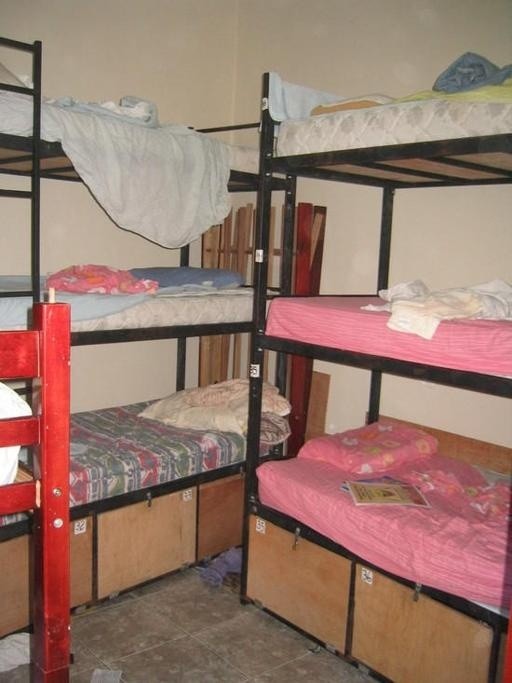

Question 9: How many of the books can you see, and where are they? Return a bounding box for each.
[341,476,421,495]
[345,483,431,507]
